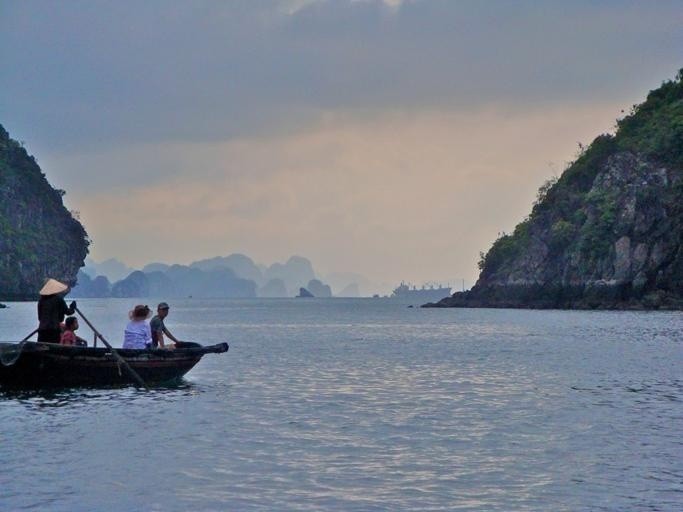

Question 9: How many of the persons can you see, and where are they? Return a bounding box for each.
[61,317,87,347]
[38,278,77,344]
[122,305,153,349]
[150,302,179,349]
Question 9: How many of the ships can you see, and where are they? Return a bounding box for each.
[393,282,452,299]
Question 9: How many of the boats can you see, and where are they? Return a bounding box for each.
[2,340,233,381]
[6,375,200,413]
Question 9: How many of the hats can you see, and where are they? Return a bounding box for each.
[38,278,68,296]
[158,302,170,310]
[128,305,153,322]
